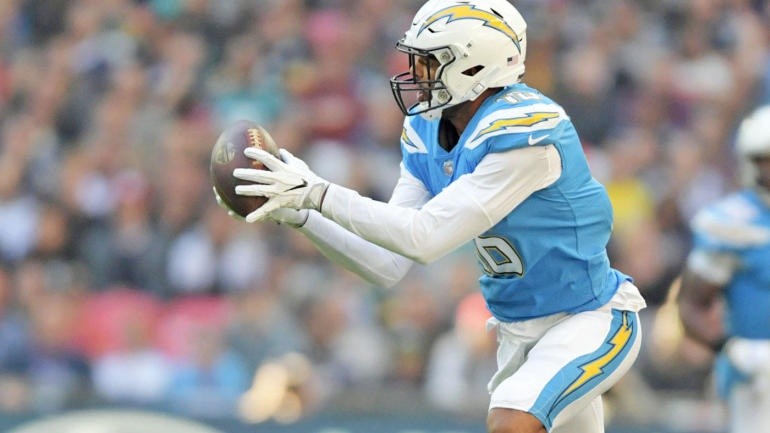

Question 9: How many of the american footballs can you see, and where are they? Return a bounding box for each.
[210,118,283,218]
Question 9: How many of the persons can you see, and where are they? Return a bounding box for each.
[0,1,504,427]
[509,0,769,432]
[212,0,647,433]
[677,105,769,431]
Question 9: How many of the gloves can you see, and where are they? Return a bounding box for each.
[214,181,311,228]
[233,143,332,224]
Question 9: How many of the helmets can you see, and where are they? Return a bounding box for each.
[389,0,526,116]
[734,105,770,191]
